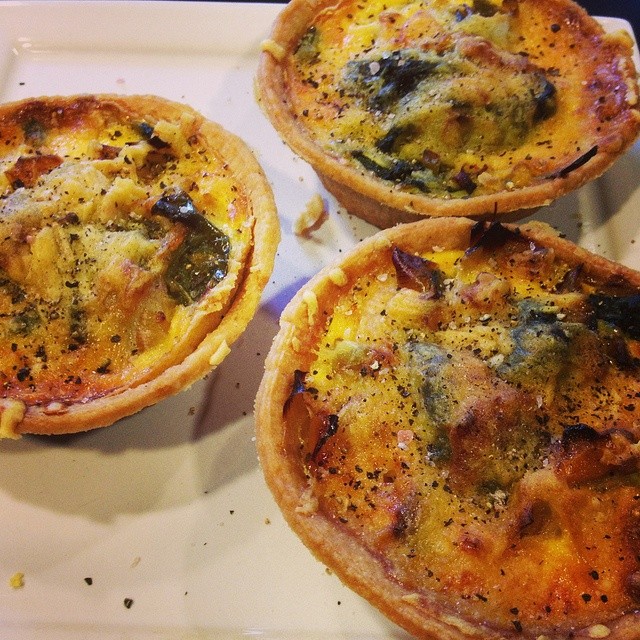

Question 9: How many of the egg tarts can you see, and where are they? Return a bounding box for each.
[250,1,640,232]
[0,92,282,438]
[253,215,640,639]
